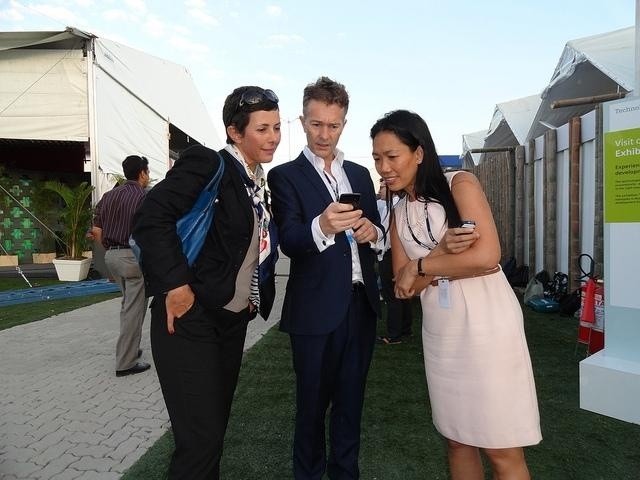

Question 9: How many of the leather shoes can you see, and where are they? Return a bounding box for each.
[115,361,151,378]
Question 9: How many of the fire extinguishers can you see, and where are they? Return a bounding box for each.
[574,254,604,353]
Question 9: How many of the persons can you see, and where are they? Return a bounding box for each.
[370,109,546,479]
[266,75,386,478]
[91,156,150,378]
[372,178,415,345]
[130,83,282,480]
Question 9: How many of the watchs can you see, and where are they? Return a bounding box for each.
[418,257,426,277]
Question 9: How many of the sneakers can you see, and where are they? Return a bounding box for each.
[137,348,143,358]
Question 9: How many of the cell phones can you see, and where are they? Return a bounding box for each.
[461,221,475,228]
[338,192,362,211]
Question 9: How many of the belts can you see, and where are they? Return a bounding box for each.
[108,243,132,250]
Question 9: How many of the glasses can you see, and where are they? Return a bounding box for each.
[228,87,281,125]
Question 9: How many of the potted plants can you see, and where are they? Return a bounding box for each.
[30,180,95,282]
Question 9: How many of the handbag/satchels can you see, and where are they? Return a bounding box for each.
[128,149,226,275]
